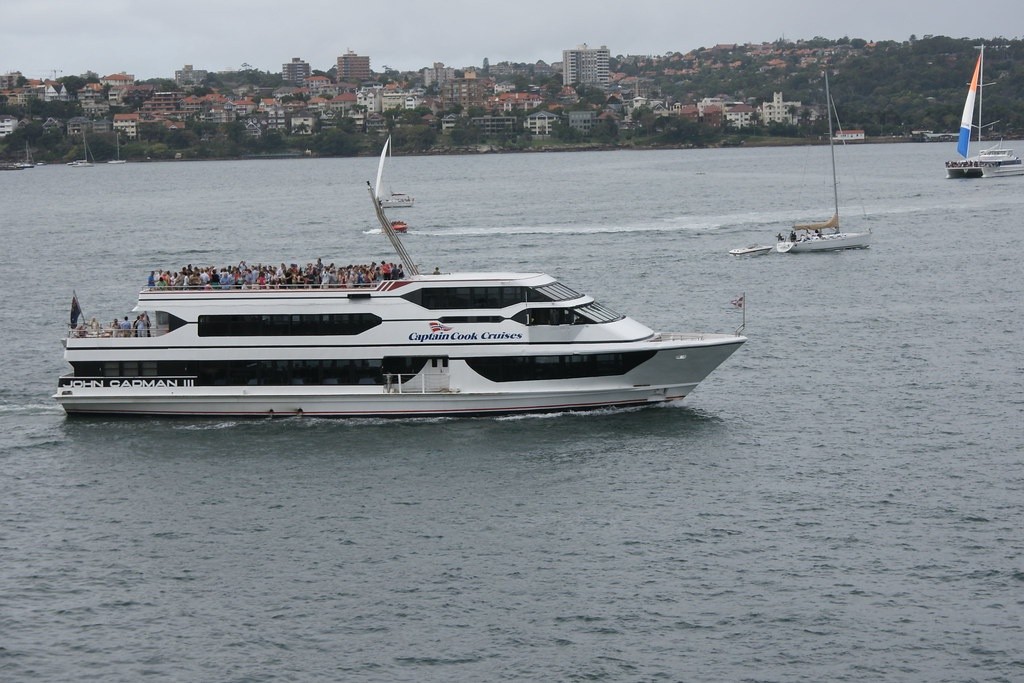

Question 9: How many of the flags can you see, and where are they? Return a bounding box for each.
[70,297,81,329]
[731,297,743,308]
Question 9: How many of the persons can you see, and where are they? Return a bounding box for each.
[433,267,440,275]
[148,258,405,290]
[112,313,151,336]
[945,161,978,167]
[79,317,99,336]
[790,230,796,242]
[806,229,819,236]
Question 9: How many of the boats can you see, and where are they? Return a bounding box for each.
[728,243,774,259]
[390,221,408,233]
[50,133,750,417]
[380,193,415,208]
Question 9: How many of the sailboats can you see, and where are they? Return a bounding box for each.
[945,44,1024,179]
[108,133,126,164]
[66,131,96,167]
[13,140,35,168]
[776,69,872,254]
